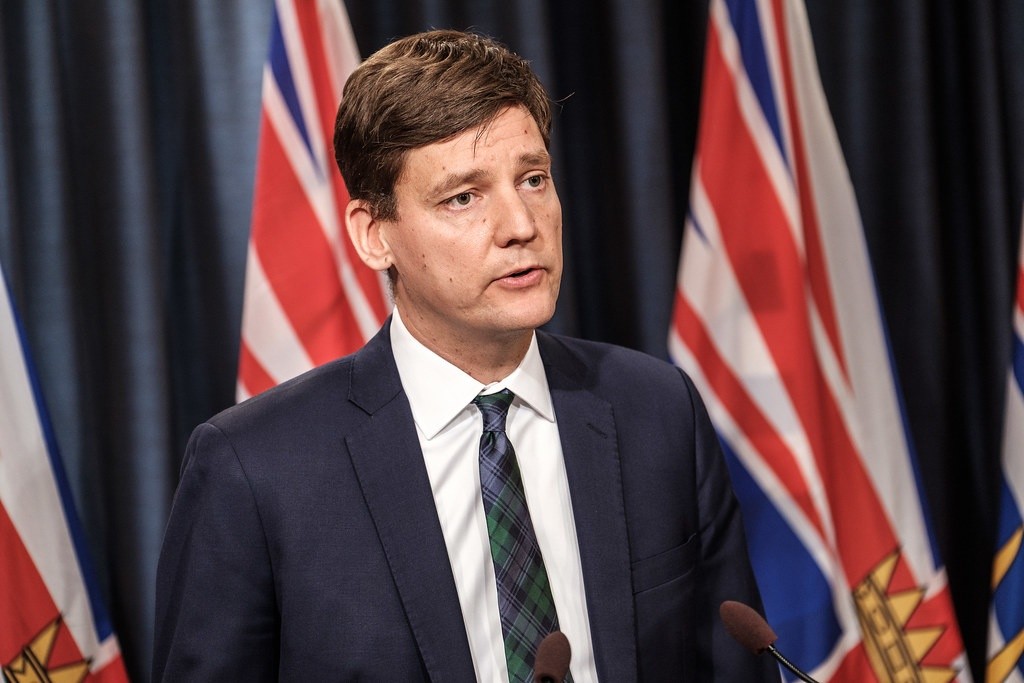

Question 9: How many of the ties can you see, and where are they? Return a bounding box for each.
[471,387,575,683]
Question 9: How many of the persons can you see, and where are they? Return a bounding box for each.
[153,32,767,683]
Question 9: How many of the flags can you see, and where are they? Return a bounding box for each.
[664,0,1024,683]
[0,266,133,683]
[233,0,403,403]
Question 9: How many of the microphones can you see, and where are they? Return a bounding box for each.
[535,631,571,683]
[720,601,817,683]
